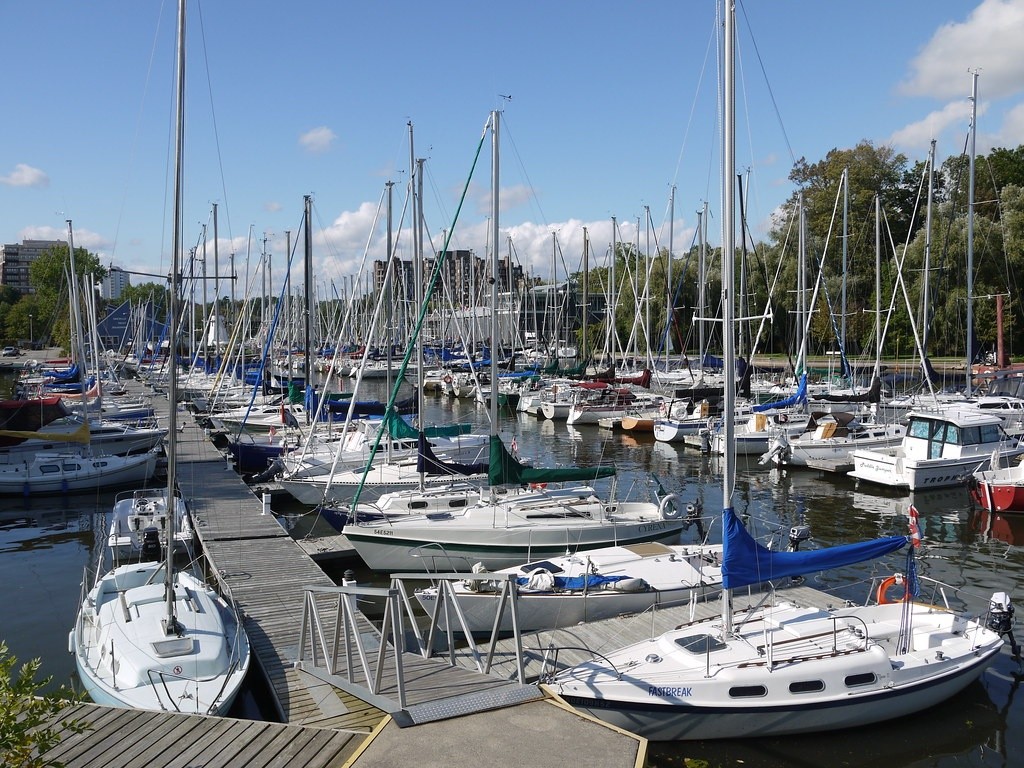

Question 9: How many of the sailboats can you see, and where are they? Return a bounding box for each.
[1,2,1024,754]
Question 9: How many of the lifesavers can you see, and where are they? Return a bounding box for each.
[876,574,912,605]
[660,495,683,519]
[532,482,547,489]
